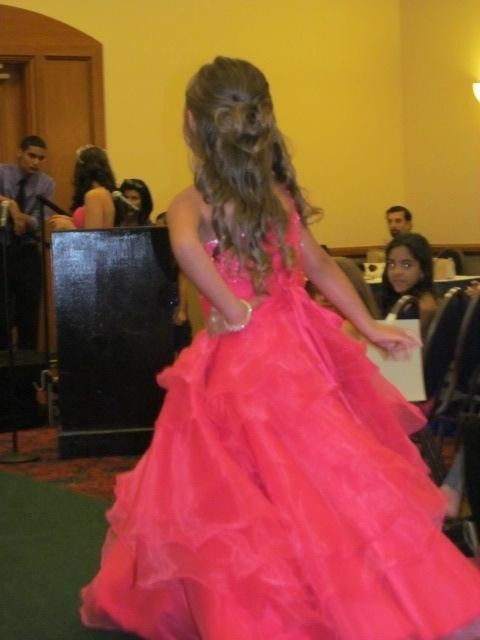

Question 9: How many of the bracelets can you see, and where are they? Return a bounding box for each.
[222,298,254,333]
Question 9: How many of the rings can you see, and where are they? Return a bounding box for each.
[211,314,216,323]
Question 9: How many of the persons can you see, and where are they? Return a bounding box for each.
[306,205,439,423]
[77,54,480,639]
[0,135,56,350]
[47,144,192,362]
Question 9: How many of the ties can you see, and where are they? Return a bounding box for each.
[14,180,26,214]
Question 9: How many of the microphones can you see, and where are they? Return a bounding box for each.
[111,190,140,213]
